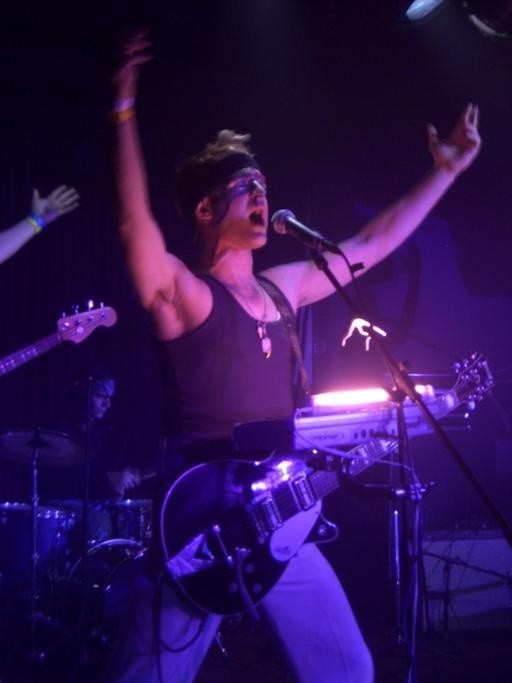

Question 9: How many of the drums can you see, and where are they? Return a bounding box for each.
[0,496,152,607]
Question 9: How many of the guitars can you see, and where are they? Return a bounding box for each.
[0,299,117,374]
[160,350,495,613]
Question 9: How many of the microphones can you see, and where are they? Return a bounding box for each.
[271,209,343,256]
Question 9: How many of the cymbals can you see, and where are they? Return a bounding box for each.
[1,429,79,462]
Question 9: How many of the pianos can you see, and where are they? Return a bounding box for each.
[230,391,471,454]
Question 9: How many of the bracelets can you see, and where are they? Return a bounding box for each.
[24,210,48,235]
[109,95,137,127]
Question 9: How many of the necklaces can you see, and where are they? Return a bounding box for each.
[230,278,277,360]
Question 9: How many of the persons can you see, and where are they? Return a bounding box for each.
[98,14,487,679]
[23,367,149,504]
[0,178,83,267]
